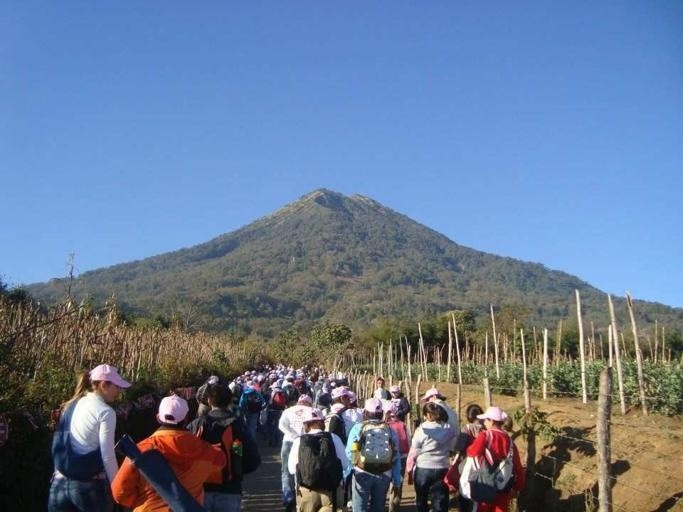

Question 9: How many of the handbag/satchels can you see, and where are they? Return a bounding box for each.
[492,456,514,490]
[53,402,103,478]
[459,455,497,501]
[328,414,346,444]
[444,460,461,494]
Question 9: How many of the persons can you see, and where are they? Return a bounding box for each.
[48,361,134,511]
[110,395,227,511]
[188,361,526,512]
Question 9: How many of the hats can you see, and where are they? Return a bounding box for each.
[159,394,190,425]
[89,363,132,389]
[476,407,507,422]
[299,386,402,422]
[422,389,439,401]
[233,365,344,388]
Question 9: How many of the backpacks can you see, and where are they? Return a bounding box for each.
[193,413,244,486]
[243,391,267,413]
[353,418,393,473]
[299,433,343,491]
[271,392,286,410]
